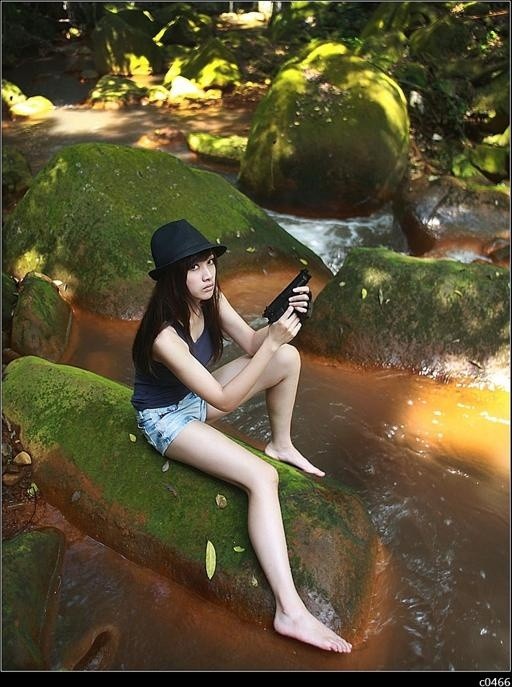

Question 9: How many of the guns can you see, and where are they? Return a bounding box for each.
[263,268,313,326]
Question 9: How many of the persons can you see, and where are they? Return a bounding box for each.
[130,218,354,655]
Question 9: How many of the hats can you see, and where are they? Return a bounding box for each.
[148,220,226,280]
